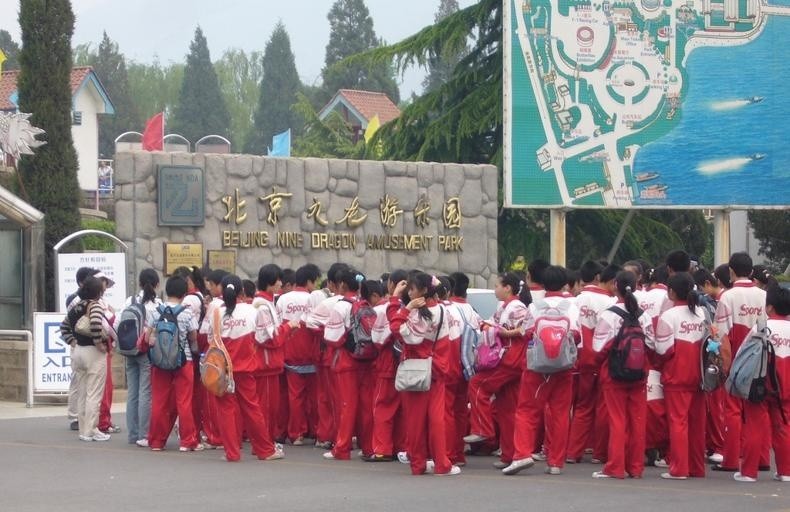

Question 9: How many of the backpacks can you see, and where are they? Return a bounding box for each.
[115,294,147,358]
[340,296,378,362]
[607,305,647,382]
[527,298,578,373]
[146,303,186,370]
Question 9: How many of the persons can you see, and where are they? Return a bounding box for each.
[99,160,113,193]
[114,263,479,474]
[463,251,790,482]
[59,267,121,442]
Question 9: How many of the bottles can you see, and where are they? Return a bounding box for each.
[199,353,205,364]
[526,341,536,351]
[707,364,720,374]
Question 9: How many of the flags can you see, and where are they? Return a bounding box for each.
[364,115,381,155]
[267,127,292,157]
[141,112,166,151]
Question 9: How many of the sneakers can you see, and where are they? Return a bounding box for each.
[136,430,304,460]
[493,445,790,482]
[315,433,486,475]
[71,421,120,442]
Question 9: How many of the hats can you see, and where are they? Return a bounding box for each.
[94,272,115,289]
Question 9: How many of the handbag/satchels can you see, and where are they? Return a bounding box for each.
[199,339,234,398]
[461,323,479,377]
[475,329,504,371]
[725,331,769,402]
[393,359,433,393]
[74,314,109,342]
[700,326,732,392]
